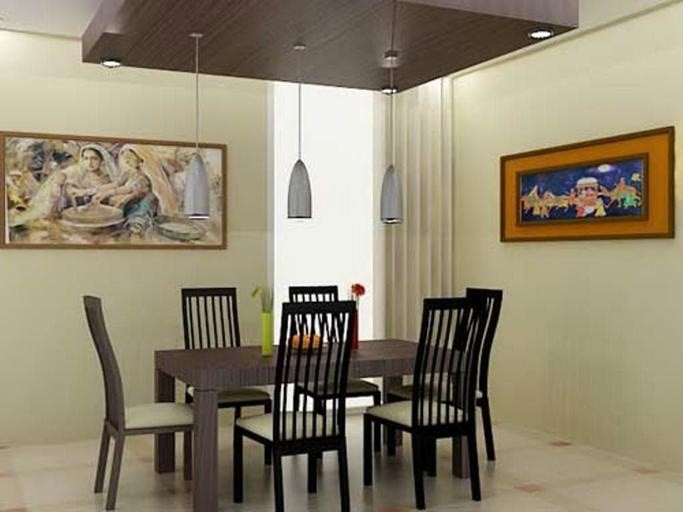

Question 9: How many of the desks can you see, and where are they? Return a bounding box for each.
[154,339,471,512]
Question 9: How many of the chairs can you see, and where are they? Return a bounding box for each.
[289,286,381,460]
[386,288,503,461]
[83,296,195,511]
[181,287,272,468]
[234,300,357,512]
[364,296,491,510]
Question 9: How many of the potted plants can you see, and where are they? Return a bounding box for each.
[251,284,272,356]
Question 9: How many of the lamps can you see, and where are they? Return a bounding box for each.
[185,32,209,218]
[381,53,403,223]
[288,44,312,219]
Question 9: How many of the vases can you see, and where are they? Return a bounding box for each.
[352,307,359,350]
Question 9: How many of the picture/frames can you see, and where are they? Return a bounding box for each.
[0,130,227,250]
[499,127,674,242]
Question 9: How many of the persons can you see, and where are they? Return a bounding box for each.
[3,135,223,247]
[522,174,644,218]
